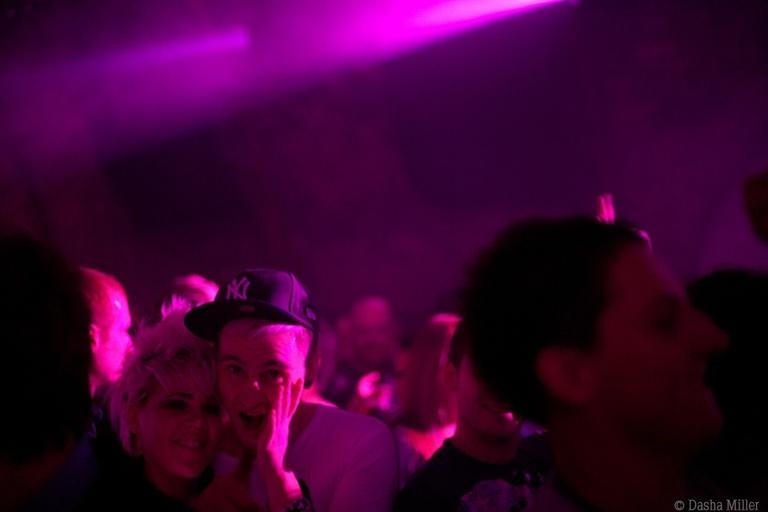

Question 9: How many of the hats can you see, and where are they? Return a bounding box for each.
[184,268,317,337]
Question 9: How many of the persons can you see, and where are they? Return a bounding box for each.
[0,169,768,512]
